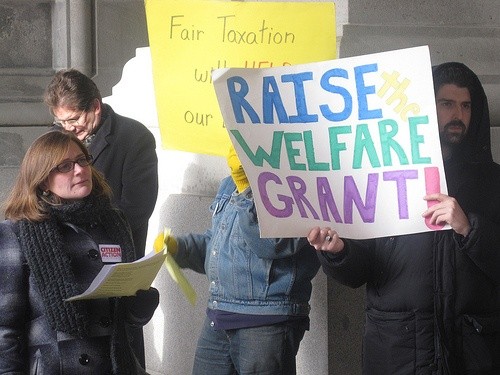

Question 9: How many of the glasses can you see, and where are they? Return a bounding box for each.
[53,108,86,127]
[53,154,93,173]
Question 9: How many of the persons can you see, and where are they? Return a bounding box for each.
[44,70,159,260]
[0,131,159,375]
[307,62,500,375]
[153,145,320,375]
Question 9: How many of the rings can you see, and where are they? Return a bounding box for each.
[325,236,332,242]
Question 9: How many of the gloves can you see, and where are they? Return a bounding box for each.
[154,233,177,260]
[227,143,250,193]
[131,287,159,318]
